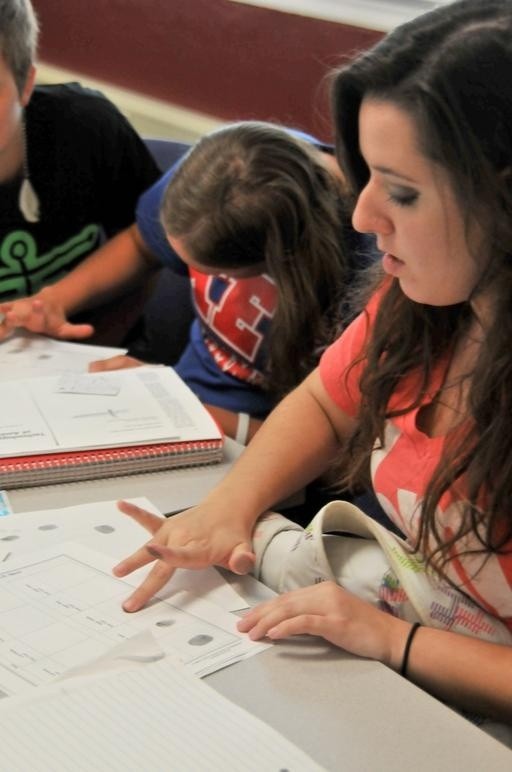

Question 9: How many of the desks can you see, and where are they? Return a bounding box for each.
[0,535,512,766]
[3,339,304,516]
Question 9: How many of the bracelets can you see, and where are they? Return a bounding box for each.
[400,623,426,674]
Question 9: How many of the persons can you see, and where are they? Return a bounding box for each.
[0,2,196,372]
[0,116,402,544]
[109,0,512,755]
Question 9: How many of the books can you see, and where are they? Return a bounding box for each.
[0,362,225,492]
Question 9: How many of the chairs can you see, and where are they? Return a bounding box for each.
[142,139,192,173]
